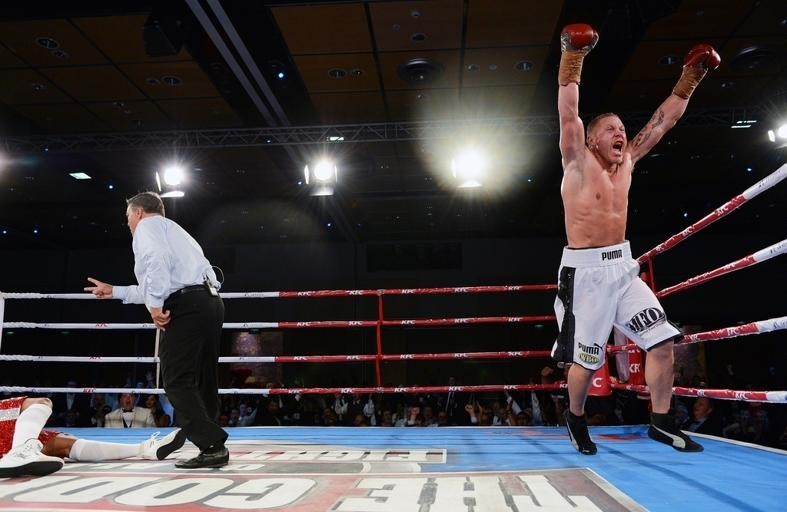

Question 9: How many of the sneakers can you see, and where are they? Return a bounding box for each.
[0,438,65,477]
[562,408,597,455]
[142,428,186,461]
[647,423,704,453]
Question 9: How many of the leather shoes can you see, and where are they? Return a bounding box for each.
[174,448,230,469]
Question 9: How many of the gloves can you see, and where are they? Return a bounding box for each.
[557,23,600,87]
[671,43,722,100]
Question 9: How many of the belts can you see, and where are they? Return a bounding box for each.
[176,287,209,295]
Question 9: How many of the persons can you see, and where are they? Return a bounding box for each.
[550,24,721,454]
[54,367,768,444]
[0,396,187,477]
[84,191,228,468]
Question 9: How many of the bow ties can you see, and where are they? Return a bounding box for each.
[123,409,133,413]
